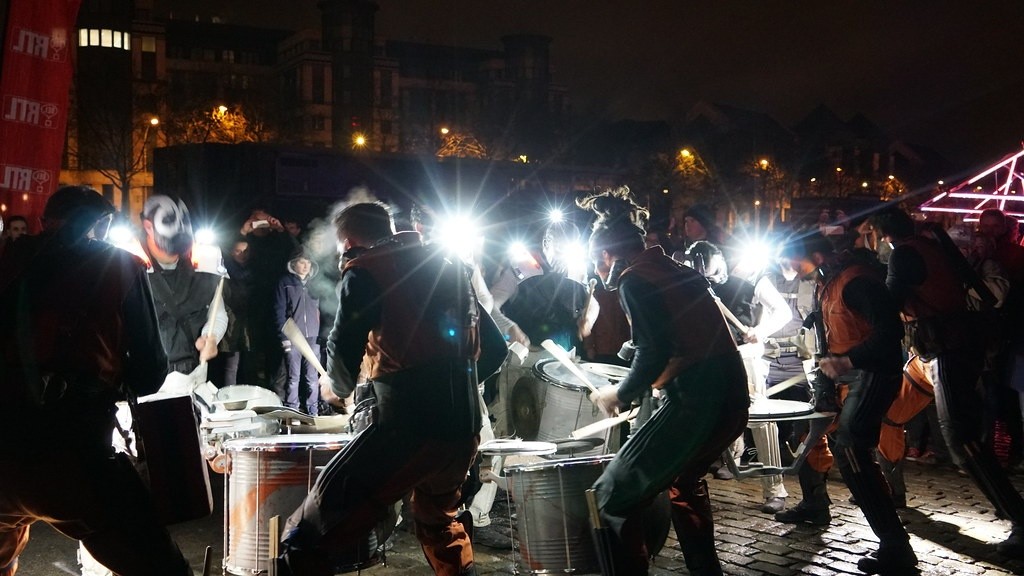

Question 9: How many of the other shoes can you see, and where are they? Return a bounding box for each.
[1014,460,1024,471]
[472,525,512,549]
[919,450,937,465]
[958,469,969,478]
[906,447,920,461]
[762,496,786,513]
[717,465,734,479]
[379,530,395,551]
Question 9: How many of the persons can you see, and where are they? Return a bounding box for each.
[863,204,1024,552]
[275,202,511,576]
[0,184,198,576]
[642,200,810,511]
[771,229,920,574]
[452,234,633,540]
[77,176,399,576]
[573,185,751,576]
[2,215,28,249]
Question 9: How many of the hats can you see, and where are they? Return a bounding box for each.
[684,202,714,232]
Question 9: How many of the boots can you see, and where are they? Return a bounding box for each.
[592,524,640,576]
[841,459,918,573]
[775,469,832,525]
[849,449,906,508]
[960,446,1024,556]
[671,496,724,576]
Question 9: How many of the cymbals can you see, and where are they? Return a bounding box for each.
[580,362,632,381]
[511,376,541,442]
[250,405,316,425]
[549,436,606,456]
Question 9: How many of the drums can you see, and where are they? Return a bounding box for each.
[528,356,615,460]
[223,432,386,576]
[503,454,612,576]
